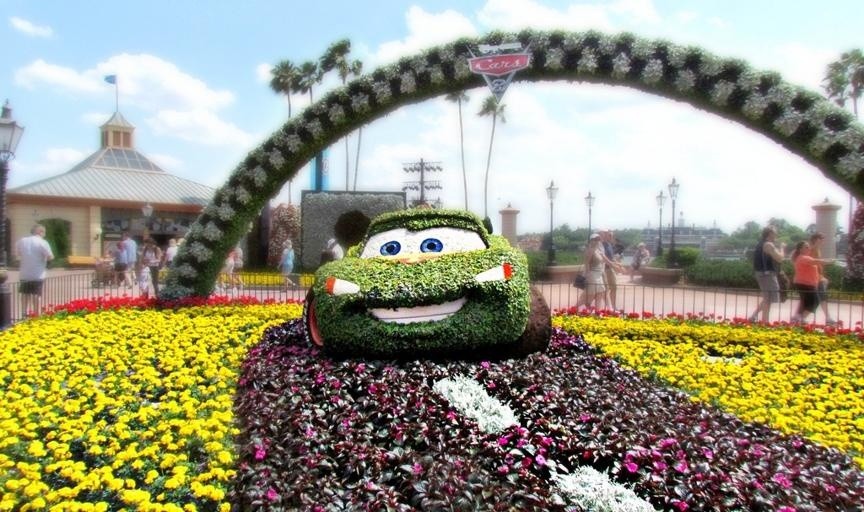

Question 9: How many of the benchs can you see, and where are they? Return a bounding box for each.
[66,255,99,271]
[639,266,684,285]
[546,264,586,284]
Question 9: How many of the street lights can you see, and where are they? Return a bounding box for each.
[544,177,561,266]
[0,97,27,336]
[667,177,680,269]
[140,199,154,242]
[656,189,667,259]
[584,190,596,248]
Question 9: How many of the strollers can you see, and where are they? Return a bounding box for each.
[90,250,117,291]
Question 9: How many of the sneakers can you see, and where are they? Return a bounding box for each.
[827,320,837,326]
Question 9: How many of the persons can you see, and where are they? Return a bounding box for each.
[807,231,837,327]
[326,238,345,259]
[597,226,627,313]
[571,234,622,309]
[14,224,57,315]
[97,224,246,299]
[749,225,785,324]
[277,240,297,293]
[790,240,838,324]
[631,241,649,271]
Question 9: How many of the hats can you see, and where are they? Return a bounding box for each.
[590,234,600,240]
[637,242,646,247]
[327,239,335,249]
[283,240,292,248]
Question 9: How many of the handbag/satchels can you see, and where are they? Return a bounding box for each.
[777,272,789,301]
[573,275,586,290]
[818,282,827,301]
